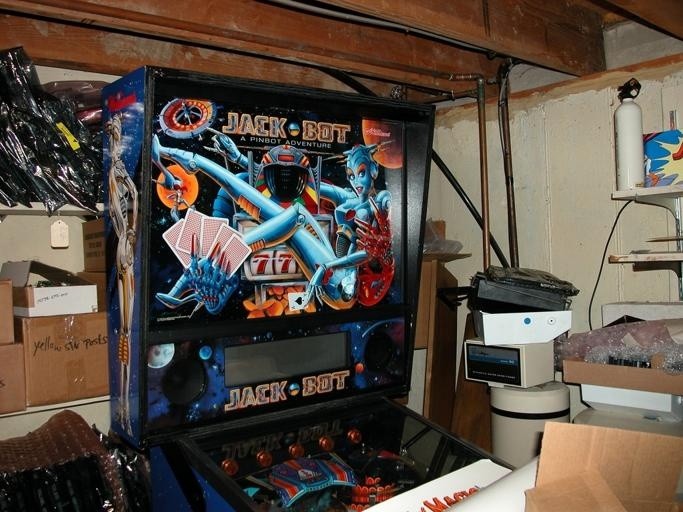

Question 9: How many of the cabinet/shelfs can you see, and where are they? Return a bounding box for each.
[0,56,438,417]
[602,185,683,327]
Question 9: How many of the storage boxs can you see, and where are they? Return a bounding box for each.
[523,317,683,512]
[0,217,123,413]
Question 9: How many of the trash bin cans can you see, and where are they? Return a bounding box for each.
[490,381,570,470]
